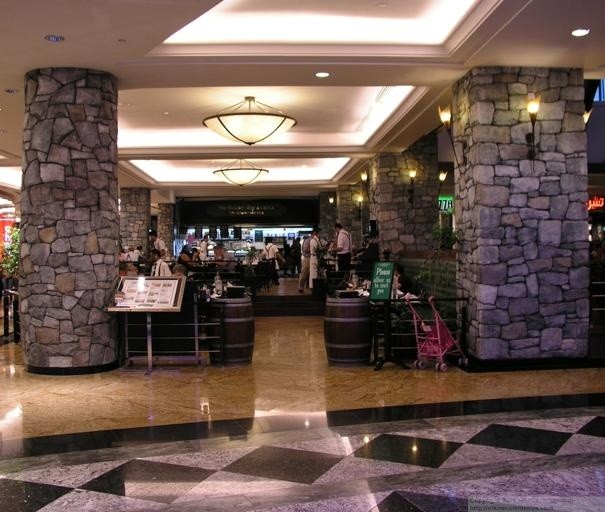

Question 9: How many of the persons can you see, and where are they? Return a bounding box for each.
[289,223,352,292]
[119,230,235,279]
[262,237,285,285]
[0,263,18,316]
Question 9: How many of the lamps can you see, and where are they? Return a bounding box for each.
[212,159,270,186]
[361,174,371,200]
[437,104,466,175]
[409,170,417,185]
[526,96,541,161]
[328,195,337,209]
[357,196,362,219]
[439,168,448,187]
[201,95,299,146]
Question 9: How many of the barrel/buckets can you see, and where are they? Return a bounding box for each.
[324,298,372,368]
[208,298,254,365]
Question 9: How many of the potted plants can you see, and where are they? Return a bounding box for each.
[0,226,21,289]
[312,246,327,300]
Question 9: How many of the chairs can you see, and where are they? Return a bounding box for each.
[119,260,274,293]
